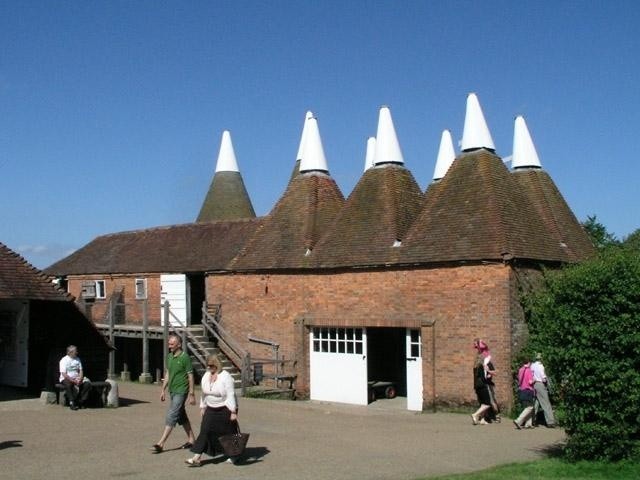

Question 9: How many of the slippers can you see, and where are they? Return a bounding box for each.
[149,444,164,453]
[184,458,201,467]
[180,441,193,449]
[225,457,235,464]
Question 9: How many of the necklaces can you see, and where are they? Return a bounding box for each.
[210,373,217,391]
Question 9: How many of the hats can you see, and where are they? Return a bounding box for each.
[535,352,543,360]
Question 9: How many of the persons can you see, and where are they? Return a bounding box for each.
[472,339,492,379]
[59,344,91,411]
[471,351,500,426]
[530,352,556,430]
[183,352,244,466]
[509,355,537,429]
[480,352,498,420]
[147,334,196,452]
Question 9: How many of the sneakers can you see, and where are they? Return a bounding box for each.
[524,425,535,429]
[547,424,556,428]
[513,420,522,429]
[78,401,85,409]
[470,413,479,425]
[478,420,490,425]
[69,402,77,410]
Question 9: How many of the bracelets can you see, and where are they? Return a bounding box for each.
[231,411,237,414]
[190,392,195,396]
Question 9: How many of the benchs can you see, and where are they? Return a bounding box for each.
[54,379,119,407]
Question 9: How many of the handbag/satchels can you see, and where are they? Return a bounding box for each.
[218,419,250,457]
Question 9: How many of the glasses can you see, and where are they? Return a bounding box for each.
[207,364,214,368]
